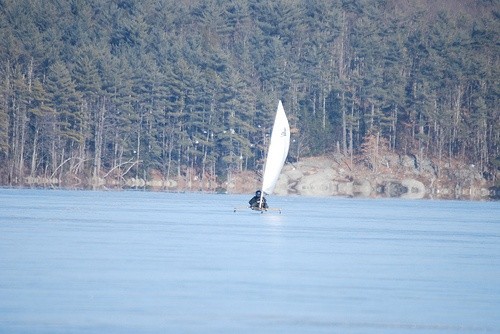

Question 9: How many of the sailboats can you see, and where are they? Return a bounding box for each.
[253,101,291,211]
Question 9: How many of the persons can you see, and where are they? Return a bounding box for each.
[249,190,269,208]
